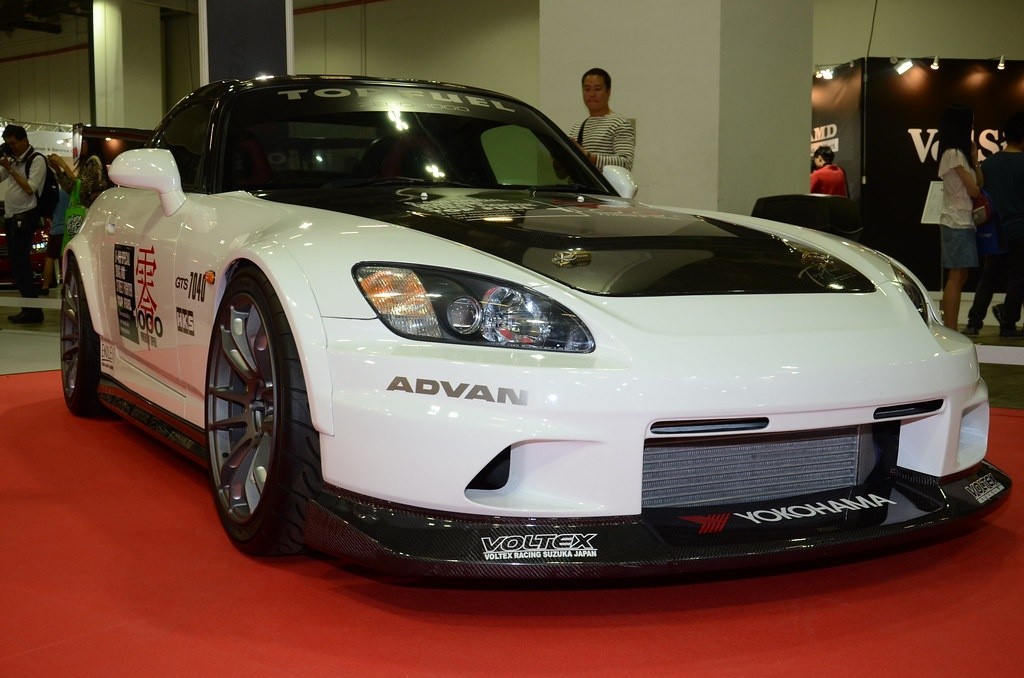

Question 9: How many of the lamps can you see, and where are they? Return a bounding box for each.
[930,55,940,70]
[814,63,841,79]
[890,56,915,75]
[993,55,1006,70]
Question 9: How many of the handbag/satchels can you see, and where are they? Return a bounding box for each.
[58,179,88,254]
[973,193,991,225]
[976,189,1000,257]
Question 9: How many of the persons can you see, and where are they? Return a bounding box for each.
[0,124,109,325]
[939,106,1024,337]
[550,68,636,193]
[811,147,849,197]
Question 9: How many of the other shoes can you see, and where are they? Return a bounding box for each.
[39,288,49,296]
[959,326,981,337]
[992,303,1018,337]
[6,310,44,324]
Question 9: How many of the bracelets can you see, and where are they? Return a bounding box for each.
[7,168,15,174]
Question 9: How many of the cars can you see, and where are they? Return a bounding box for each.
[61,74,1011,584]
[0,200,61,288]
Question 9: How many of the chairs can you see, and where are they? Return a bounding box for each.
[349,135,444,178]
[222,130,273,187]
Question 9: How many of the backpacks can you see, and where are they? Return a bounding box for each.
[24,151,60,216]
